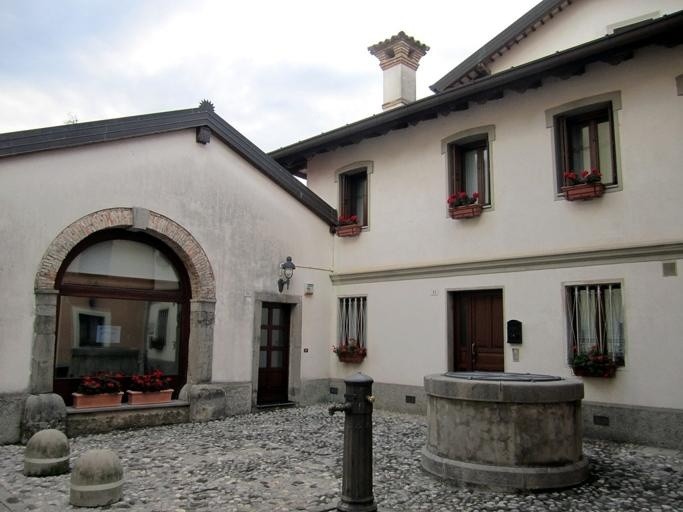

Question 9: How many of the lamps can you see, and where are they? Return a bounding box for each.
[278,257,295,295]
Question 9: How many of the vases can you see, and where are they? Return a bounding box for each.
[333,225,361,237]
[123,383,174,406]
[71,390,124,408]
[335,353,365,365]
[573,366,618,378]
[557,183,606,200]
[448,204,483,219]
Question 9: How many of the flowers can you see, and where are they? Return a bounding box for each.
[335,212,360,227]
[126,368,170,391]
[571,344,626,368]
[446,191,479,208]
[562,168,606,183]
[73,369,125,396]
[332,335,365,356]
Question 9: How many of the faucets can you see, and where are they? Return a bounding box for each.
[328,402,349,417]
